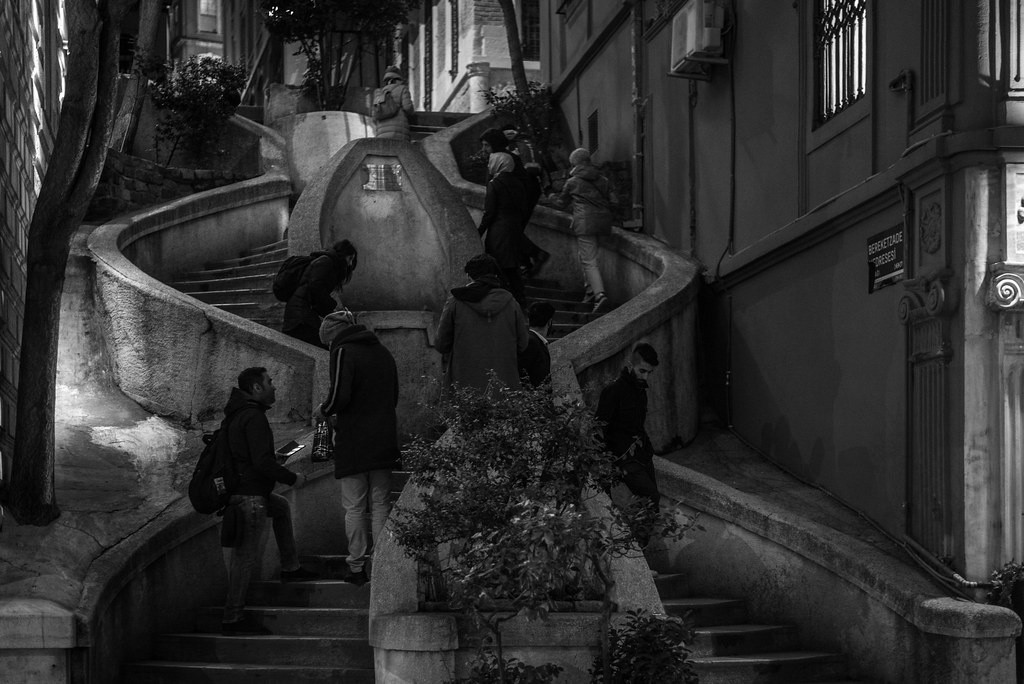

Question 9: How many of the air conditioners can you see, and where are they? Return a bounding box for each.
[670,1,733,78]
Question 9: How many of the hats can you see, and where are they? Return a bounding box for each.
[319,310,355,342]
[383,65,404,80]
[480,128,510,153]
[569,148,590,165]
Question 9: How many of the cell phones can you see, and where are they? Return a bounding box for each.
[284,444,306,457]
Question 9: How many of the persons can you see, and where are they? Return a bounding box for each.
[374,65,416,140]
[516,301,556,411]
[216,365,320,637]
[594,342,663,578]
[282,239,358,350]
[477,152,528,310]
[506,141,549,277]
[434,251,530,407]
[548,149,615,314]
[312,308,399,587]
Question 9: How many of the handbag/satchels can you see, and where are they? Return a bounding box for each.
[311,416,333,463]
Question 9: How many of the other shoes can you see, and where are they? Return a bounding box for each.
[221,622,255,637]
[344,569,369,582]
[581,290,595,303]
[591,294,608,314]
[280,566,318,582]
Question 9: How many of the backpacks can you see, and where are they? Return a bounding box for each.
[188,404,262,515]
[373,84,405,121]
[272,255,336,303]
